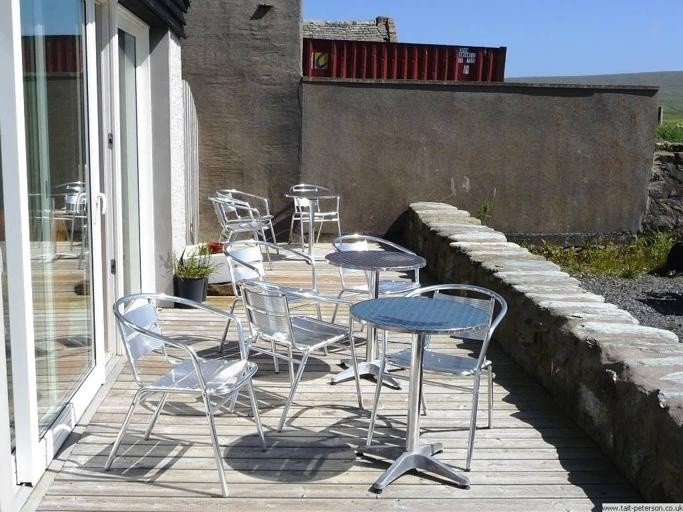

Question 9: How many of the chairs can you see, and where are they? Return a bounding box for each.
[27,180,86,271]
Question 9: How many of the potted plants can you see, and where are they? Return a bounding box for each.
[74,280,90,295]
